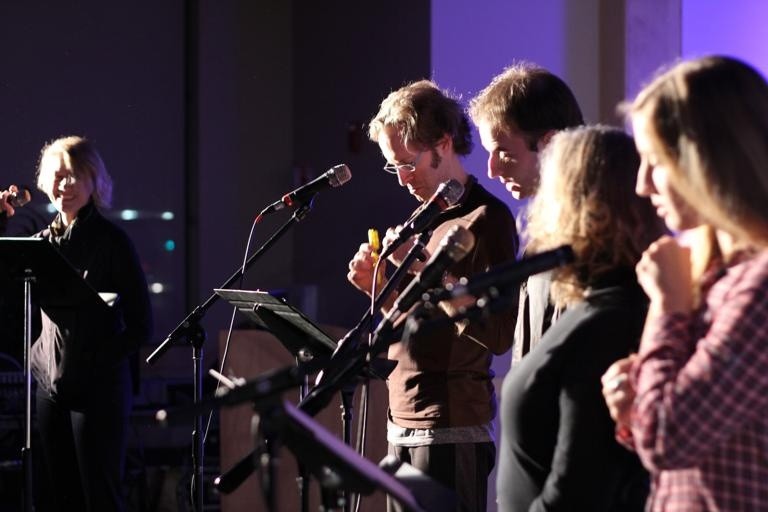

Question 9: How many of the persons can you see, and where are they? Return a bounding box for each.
[346,80,520,512]
[600,55,768,512]
[467,62,585,366]
[0,136,154,512]
[496,125,674,512]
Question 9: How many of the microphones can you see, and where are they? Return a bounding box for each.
[375,223,474,334]
[442,243,576,303]
[8,189,30,209]
[379,177,466,260]
[262,164,353,214]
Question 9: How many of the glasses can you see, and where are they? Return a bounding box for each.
[383,146,427,175]
